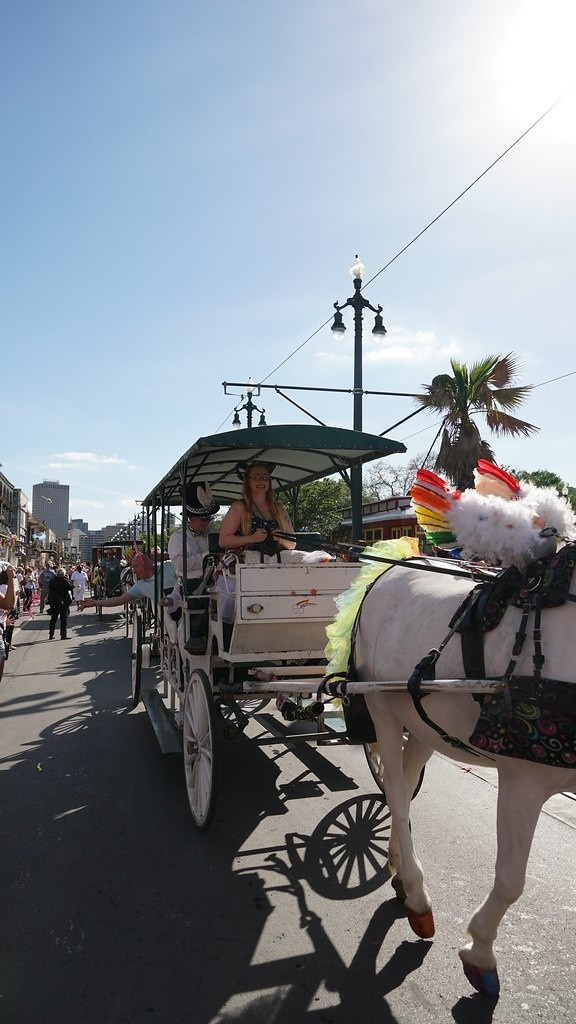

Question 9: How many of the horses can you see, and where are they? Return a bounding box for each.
[352,548,576,995]
[120,566,140,624]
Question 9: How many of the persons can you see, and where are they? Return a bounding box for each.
[168,514,213,637]
[160,577,183,626]
[100,549,119,576]
[0,561,94,682]
[77,553,178,611]
[217,464,331,625]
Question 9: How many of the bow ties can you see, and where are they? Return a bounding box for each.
[193,532,206,538]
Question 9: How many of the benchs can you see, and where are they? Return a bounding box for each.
[206,531,322,651]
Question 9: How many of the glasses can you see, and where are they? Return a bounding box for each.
[198,515,210,522]
[248,476,269,481]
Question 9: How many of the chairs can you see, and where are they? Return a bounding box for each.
[164,586,183,689]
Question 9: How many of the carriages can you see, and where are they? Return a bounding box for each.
[88,540,141,624]
[130,422,576,998]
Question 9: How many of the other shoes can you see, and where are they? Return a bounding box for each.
[61,636,71,640]
[49,637,55,640]
[7,644,17,650]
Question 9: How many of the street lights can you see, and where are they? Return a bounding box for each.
[232,376,268,429]
[331,254,388,548]
[109,505,147,557]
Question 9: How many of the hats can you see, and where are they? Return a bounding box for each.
[179,481,220,517]
[28,569,33,572]
